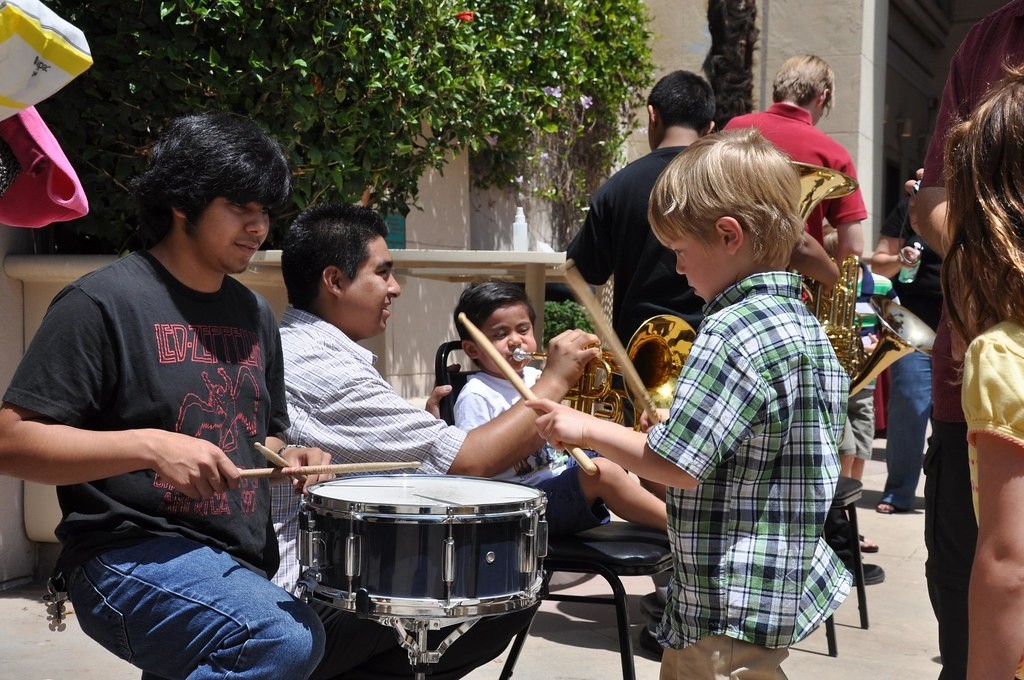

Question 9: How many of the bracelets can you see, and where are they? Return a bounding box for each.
[278,445,306,458]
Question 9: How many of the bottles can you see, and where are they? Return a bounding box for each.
[898,242,921,283]
[512,207,528,251]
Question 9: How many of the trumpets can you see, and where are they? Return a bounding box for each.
[510,312,701,462]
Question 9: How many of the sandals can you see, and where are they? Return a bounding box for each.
[876,501,900,514]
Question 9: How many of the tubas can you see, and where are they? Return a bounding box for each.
[784,156,936,421]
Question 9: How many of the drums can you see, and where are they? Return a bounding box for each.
[293,474,547,621]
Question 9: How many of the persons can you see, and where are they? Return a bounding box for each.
[871,196,942,513]
[0,110,540,680]
[942,64,1024,680]
[453,277,669,537]
[824,224,886,587]
[545,69,841,428]
[515,128,854,680]
[272,201,600,593]
[0,114,76,228]
[721,54,867,262]
[908,1,1023,680]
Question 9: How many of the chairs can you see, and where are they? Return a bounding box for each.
[821,477,868,657]
[434,341,672,680]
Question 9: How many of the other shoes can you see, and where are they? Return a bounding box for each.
[859,538,879,553]
[639,628,664,655]
[843,563,884,585]
